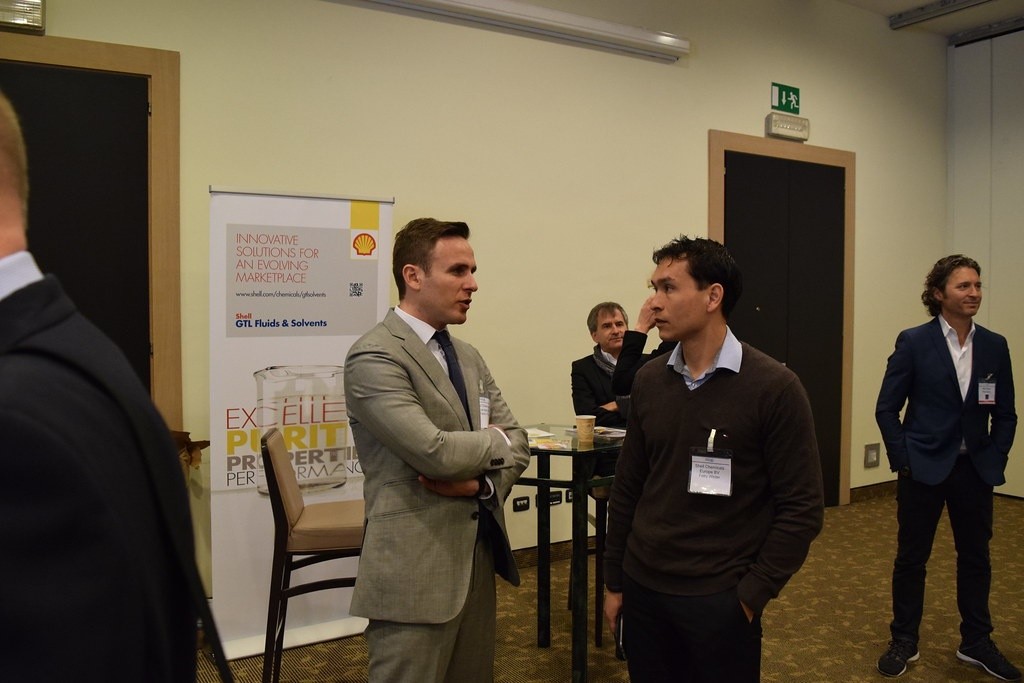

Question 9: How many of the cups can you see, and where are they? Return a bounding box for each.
[576,415,596,443]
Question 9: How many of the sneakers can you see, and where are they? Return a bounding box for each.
[877,640,920,677]
[956,639,1022,681]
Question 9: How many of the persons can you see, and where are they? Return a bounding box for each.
[569,236,826,682]
[875,253,1024,683]
[341,218,532,683]
[2,95,206,683]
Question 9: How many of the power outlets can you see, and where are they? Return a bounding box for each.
[865,443,880,467]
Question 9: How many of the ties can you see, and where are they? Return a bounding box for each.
[431,330,474,431]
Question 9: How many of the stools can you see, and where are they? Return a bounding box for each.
[257,428,365,683]
[568,478,611,648]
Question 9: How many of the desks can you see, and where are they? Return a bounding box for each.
[520,424,627,683]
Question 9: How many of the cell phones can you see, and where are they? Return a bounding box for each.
[620,614,627,661]
